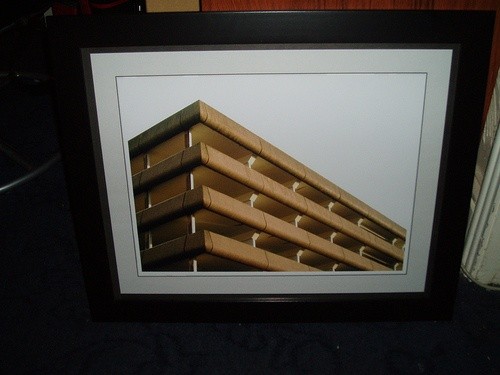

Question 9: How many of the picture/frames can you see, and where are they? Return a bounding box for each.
[43,7,497,326]
